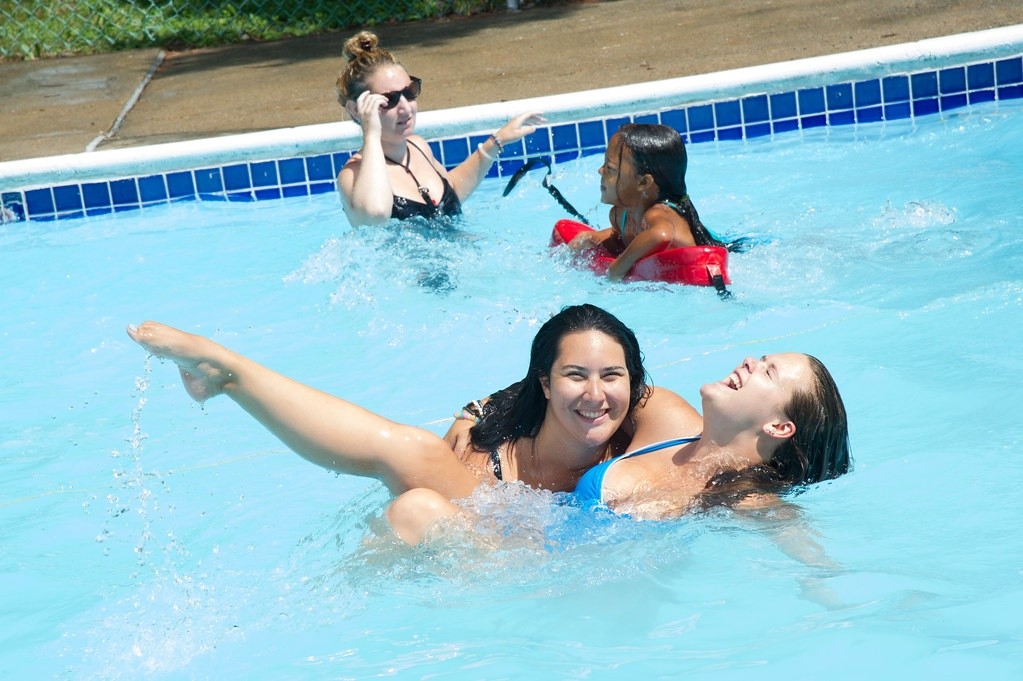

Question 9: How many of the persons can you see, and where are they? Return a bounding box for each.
[568,123,714,276]
[126,322,850,547]
[335,30,547,229]
[442,303,655,492]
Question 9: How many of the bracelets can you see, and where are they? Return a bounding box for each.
[478,143,496,161]
[491,134,504,153]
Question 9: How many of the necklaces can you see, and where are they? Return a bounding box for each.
[385,146,435,211]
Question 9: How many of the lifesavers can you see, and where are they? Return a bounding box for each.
[548,218,733,287]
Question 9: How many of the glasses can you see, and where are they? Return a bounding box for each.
[378,75,422,110]
